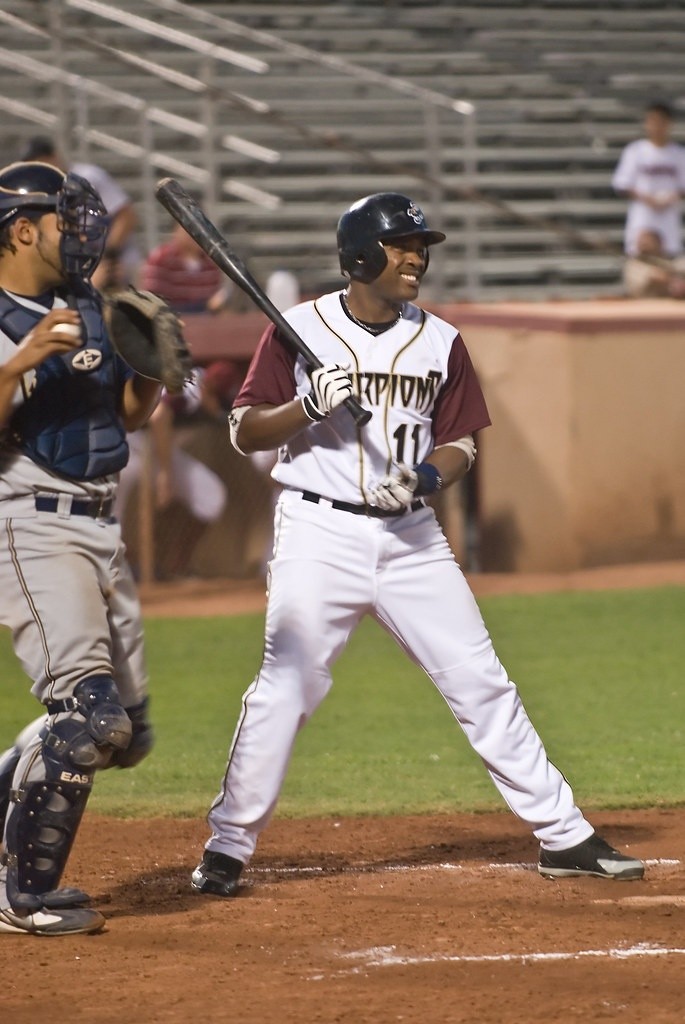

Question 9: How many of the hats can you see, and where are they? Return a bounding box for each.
[22,136,56,161]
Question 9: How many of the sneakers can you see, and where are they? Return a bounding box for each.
[0,904,106,935]
[538,832,645,881]
[191,849,244,896]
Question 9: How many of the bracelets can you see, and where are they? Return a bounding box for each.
[412,463,442,496]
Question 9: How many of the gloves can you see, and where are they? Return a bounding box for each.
[301,363,356,422]
[364,461,442,511]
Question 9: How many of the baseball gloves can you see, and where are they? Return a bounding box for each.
[104,284,198,389]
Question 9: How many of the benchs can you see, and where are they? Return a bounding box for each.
[0,0,685,303]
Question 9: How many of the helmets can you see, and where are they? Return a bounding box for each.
[0,161,86,222]
[337,193,446,284]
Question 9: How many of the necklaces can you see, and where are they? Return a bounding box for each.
[343,290,402,333]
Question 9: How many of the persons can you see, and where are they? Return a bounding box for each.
[0,161,165,937]
[72,163,278,533]
[612,102,685,298]
[190,192,645,898]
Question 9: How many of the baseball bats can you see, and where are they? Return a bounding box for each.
[152,177,372,428]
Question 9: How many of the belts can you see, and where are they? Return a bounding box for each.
[35,496,117,519]
[301,490,424,518]
